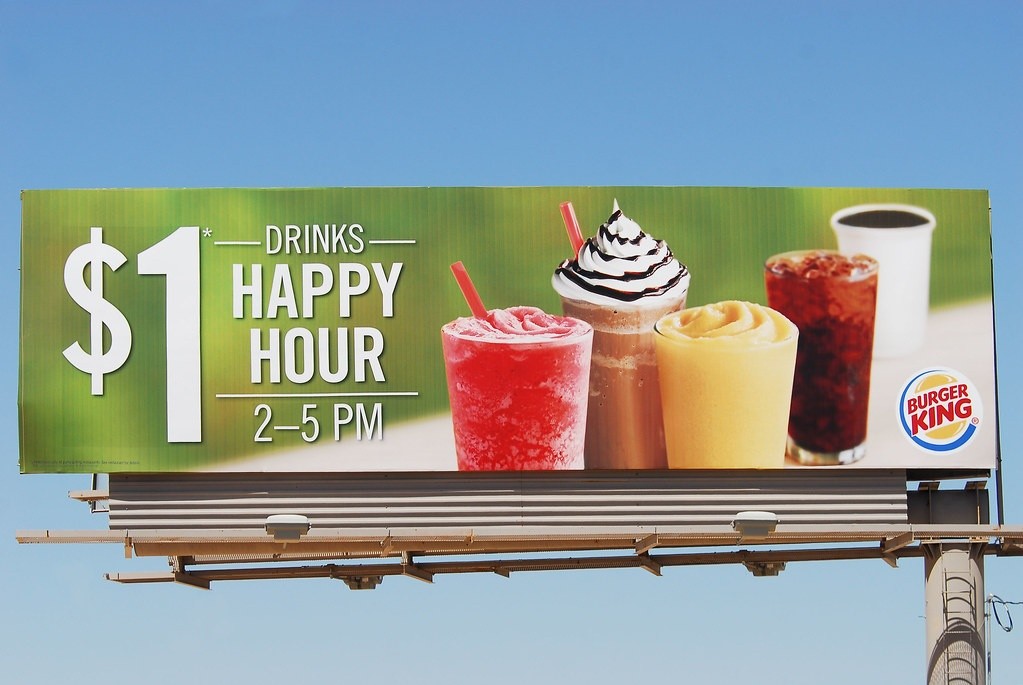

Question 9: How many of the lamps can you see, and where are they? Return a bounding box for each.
[343,575,384,590]
[263,514,312,544]
[744,561,787,577]
[731,511,780,539]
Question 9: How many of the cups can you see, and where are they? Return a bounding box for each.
[560,289,687,469]
[654,311,799,469]
[441,317,594,470]
[831,204,936,356]
[765,250,879,466]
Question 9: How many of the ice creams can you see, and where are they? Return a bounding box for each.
[550,196,691,308]
